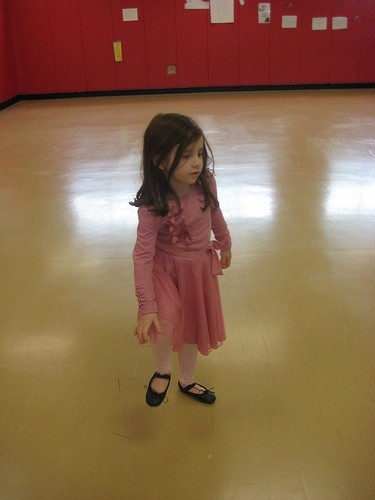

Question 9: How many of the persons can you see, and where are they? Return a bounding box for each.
[132,113,231,406]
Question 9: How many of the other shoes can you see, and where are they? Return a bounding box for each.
[145,369,172,406]
[177,378,216,404]
